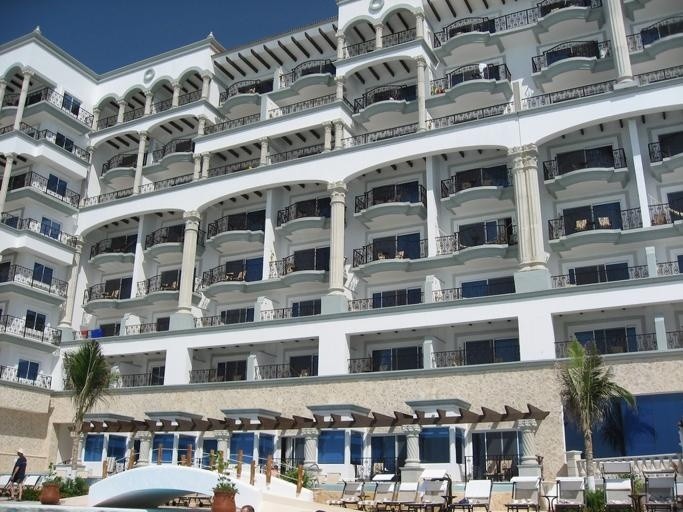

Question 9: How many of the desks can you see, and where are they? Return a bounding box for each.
[627,493,646,511]
[442,495,456,509]
[539,495,558,511]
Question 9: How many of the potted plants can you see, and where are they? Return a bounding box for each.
[211,472,238,512]
[37,473,59,505]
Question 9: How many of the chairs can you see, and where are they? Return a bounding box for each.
[357,479,398,511]
[602,477,636,511]
[553,480,587,511]
[642,470,679,511]
[504,480,539,511]
[382,482,420,511]
[404,477,453,511]
[448,479,493,511]
[0,473,56,500]
[326,477,366,509]
[82,213,683,302]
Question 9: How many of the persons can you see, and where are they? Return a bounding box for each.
[8,448,27,500]
[240,504,255,512]
